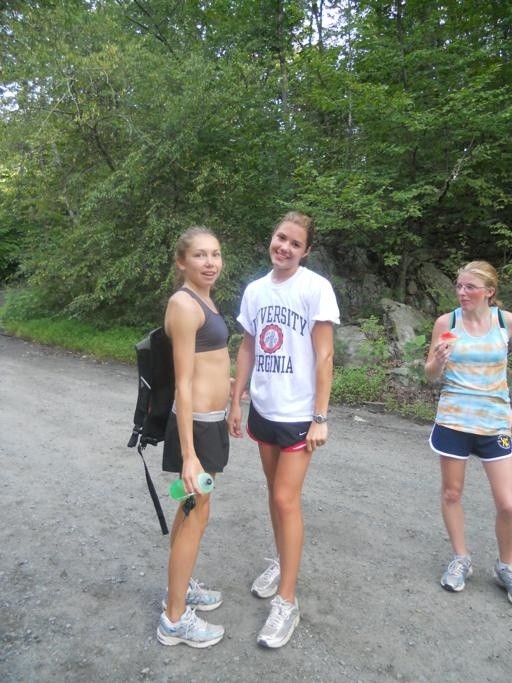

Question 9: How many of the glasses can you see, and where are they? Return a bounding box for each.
[455,282,490,292]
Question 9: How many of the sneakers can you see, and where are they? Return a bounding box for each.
[155,604,227,650]
[161,576,223,612]
[439,554,474,592]
[492,556,512,604]
[249,556,282,599]
[256,594,300,650]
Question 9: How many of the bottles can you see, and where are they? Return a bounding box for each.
[169,472,215,502]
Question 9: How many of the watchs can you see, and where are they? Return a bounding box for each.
[312,414,328,423]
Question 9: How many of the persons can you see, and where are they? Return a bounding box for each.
[226,209,340,648]
[424,260,512,605]
[156,226,248,646]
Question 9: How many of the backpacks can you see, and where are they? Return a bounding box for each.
[127,325,168,453]
